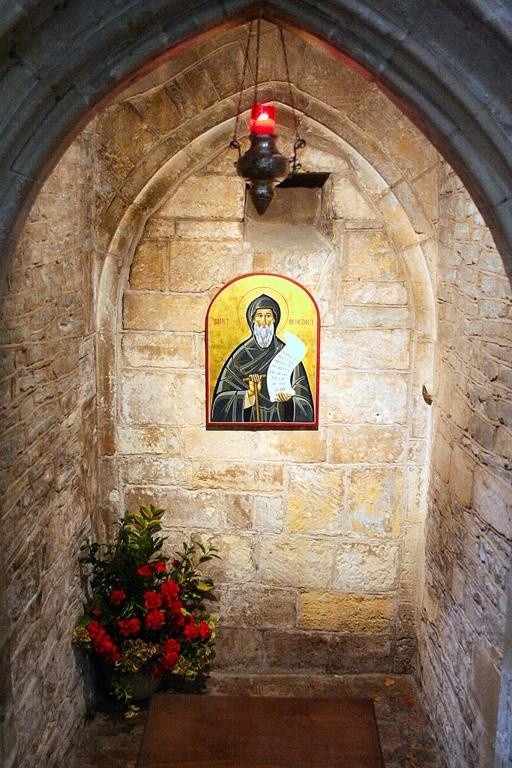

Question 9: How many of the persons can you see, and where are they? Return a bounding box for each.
[212,292,315,423]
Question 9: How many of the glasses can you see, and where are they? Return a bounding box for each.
[229,0,306,217]
